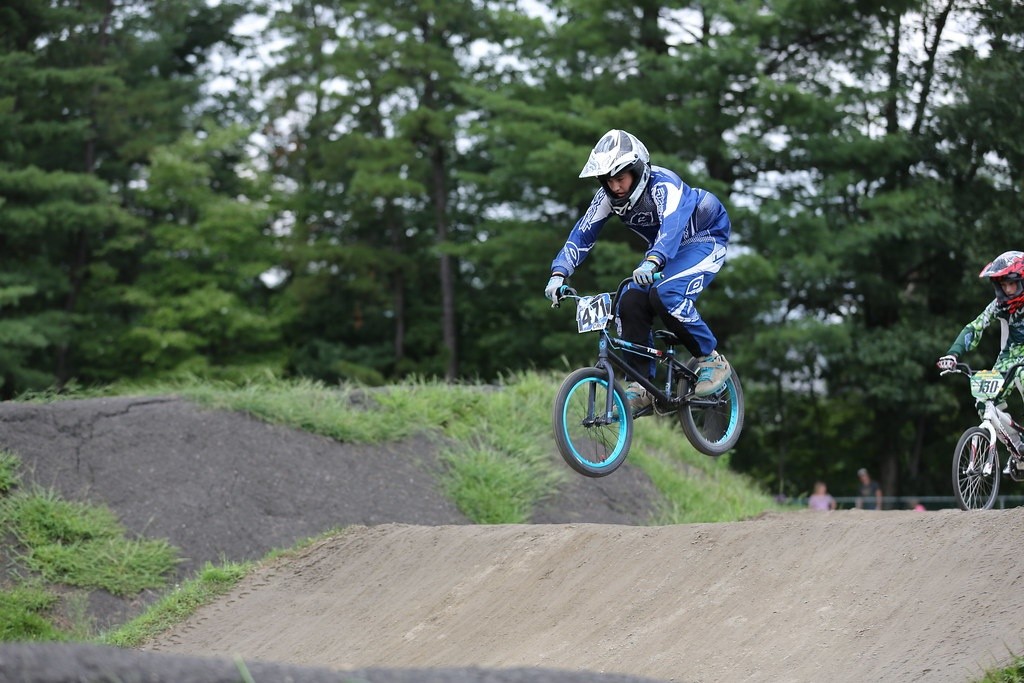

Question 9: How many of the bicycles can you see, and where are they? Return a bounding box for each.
[939,358,1024,511]
[552,271,744,478]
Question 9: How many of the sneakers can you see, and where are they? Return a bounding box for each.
[695,353,732,397]
[609,380,656,418]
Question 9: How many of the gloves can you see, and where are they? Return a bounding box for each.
[545,274,565,308]
[633,260,659,285]
[936,354,957,371]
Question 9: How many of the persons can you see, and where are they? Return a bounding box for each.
[936,248,1024,476]
[544,127,732,422]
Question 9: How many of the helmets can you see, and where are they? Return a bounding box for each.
[578,129,651,216]
[979,251,1024,314]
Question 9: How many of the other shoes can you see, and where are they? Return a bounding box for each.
[1002,453,1016,474]
